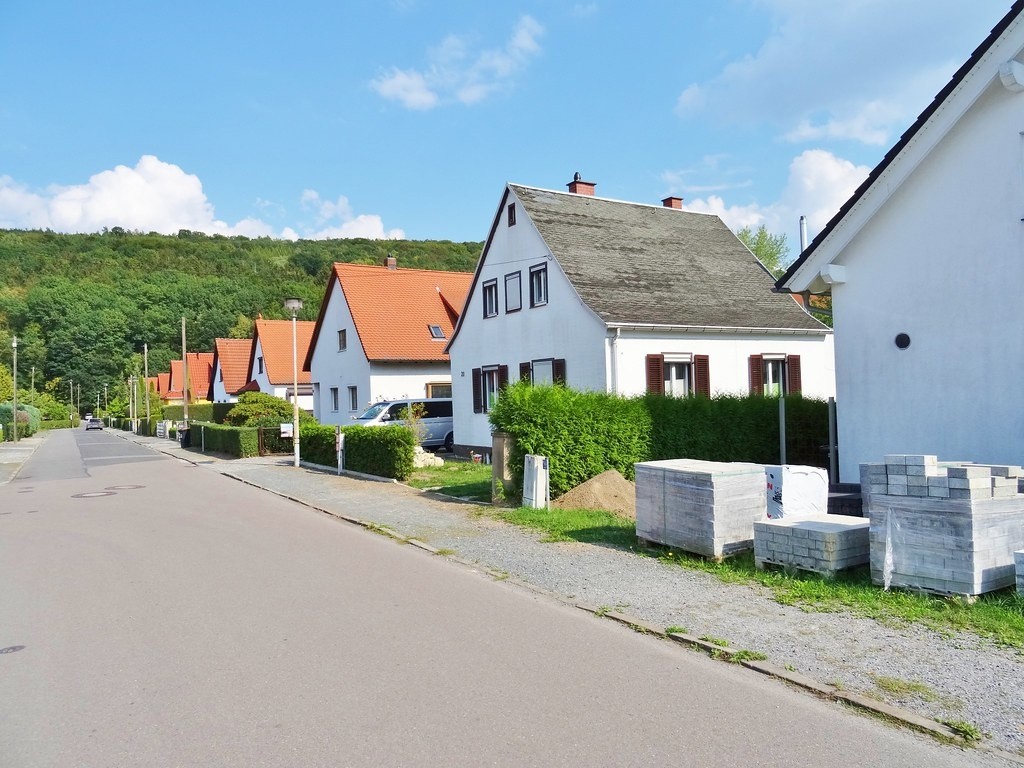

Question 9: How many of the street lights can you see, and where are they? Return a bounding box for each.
[283,296,304,467]
[103,383,108,416]
[76,384,81,413]
[69,380,73,419]
[12,341,18,442]
[96,391,100,417]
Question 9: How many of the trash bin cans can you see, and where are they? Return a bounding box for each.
[178,428,191,449]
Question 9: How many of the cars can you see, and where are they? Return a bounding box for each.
[86,418,104,430]
[84,413,93,420]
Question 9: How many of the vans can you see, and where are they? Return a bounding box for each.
[344,398,453,452]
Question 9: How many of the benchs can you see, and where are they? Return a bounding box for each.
[157,420,172,439]
